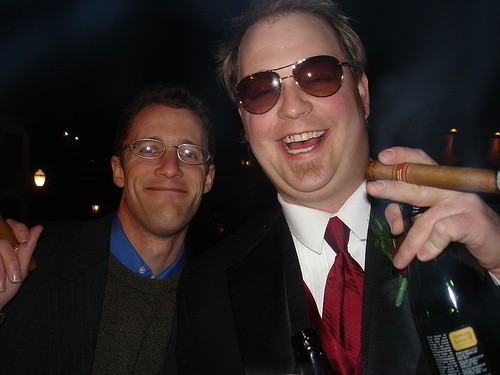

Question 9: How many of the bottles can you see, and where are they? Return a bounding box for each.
[400,202,500,375]
[290,328,338,375]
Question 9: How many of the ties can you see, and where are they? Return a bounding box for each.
[321,216,364,375]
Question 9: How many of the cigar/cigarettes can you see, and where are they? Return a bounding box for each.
[365,159,500,196]
[0,214,38,272]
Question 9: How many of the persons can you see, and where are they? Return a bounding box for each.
[176,1,500,375]
[0,85,214,375]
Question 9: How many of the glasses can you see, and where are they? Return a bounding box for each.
[235,55,357,115]
[121,139,213,165]
